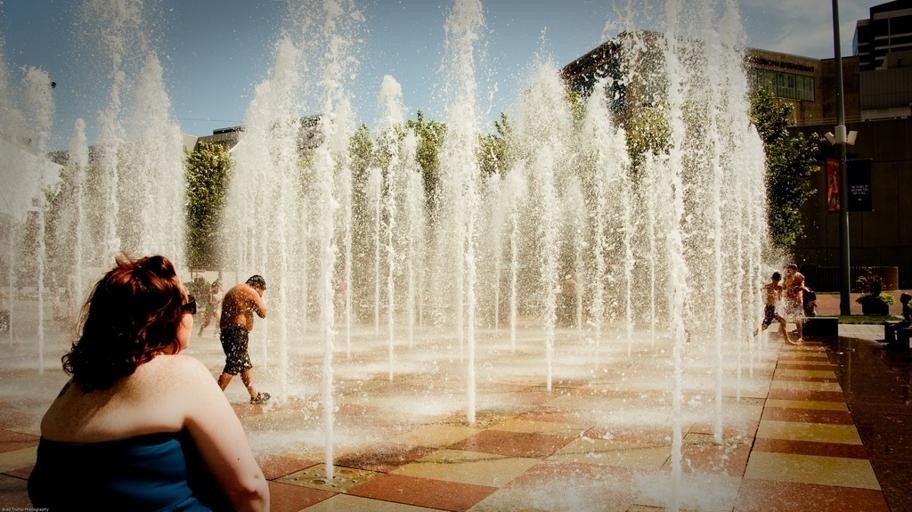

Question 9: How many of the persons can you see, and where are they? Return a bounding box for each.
[218,274,272,405]
[27,257,271,512]
[781,264,811,339]
[803,288,817,316]
[745,271,797,346]
[198,280,220,337]
[562,267,576,326]
[887,292,912,345]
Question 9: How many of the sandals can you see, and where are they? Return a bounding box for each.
[250,392,272,404]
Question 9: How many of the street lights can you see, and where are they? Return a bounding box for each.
[823,124,858,317]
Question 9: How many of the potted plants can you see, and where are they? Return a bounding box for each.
[854,265,897,317]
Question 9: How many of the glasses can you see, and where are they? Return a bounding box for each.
[170,294,198,316]
